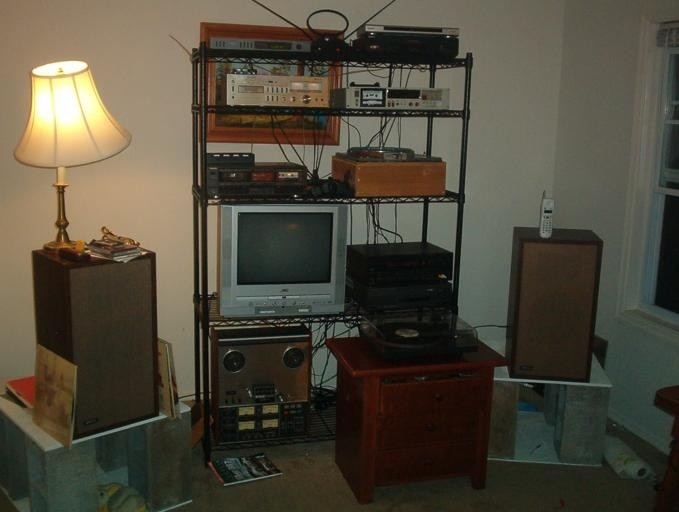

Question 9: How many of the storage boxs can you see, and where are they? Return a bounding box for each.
[330,155,446,198]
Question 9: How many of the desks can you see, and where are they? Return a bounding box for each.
[653,385,679,512]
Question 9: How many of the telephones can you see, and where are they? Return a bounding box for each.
[539,191,555,238]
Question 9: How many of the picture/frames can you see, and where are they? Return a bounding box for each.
[199,22,345,145]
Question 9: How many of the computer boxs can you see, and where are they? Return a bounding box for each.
[211,325,312,408]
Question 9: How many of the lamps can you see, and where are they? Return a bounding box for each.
[11,61,133,254]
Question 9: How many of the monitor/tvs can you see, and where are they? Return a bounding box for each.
[220,204,348,321]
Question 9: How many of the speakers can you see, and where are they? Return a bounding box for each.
[31,245,159,440]
[504,224,603,385]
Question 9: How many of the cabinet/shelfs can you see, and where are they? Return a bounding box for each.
[483,348,615,468]
[190,38,474,471]
[0,387,194,512]
[322,331,512,506]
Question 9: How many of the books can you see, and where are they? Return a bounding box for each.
[86,239,149,263]
[207,452,283,486]
